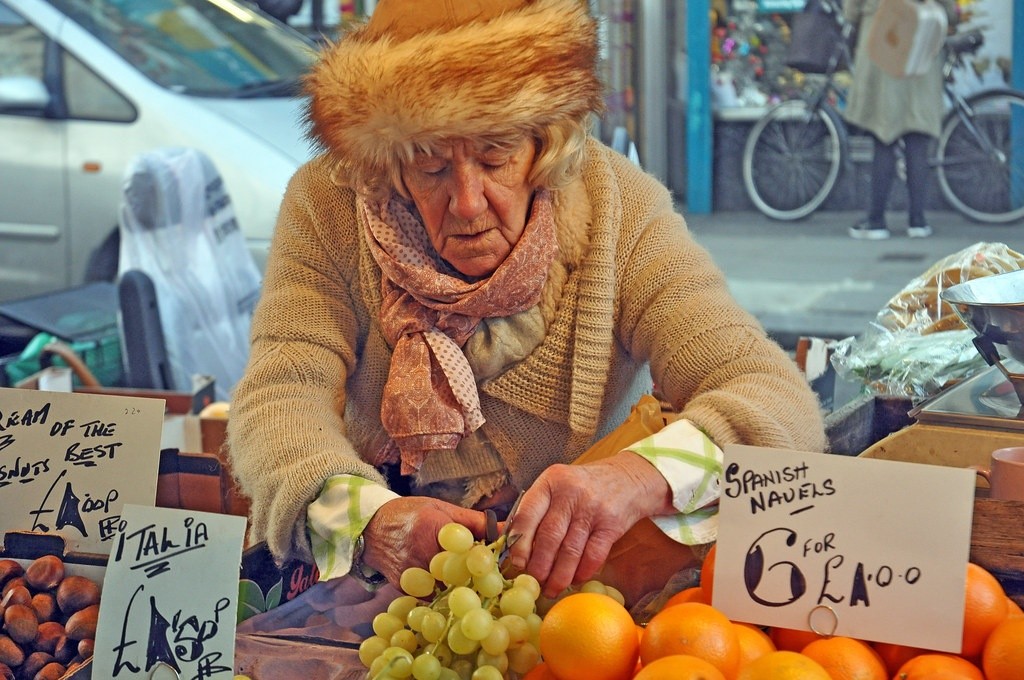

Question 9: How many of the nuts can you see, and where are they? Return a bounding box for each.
[0,555,99,680]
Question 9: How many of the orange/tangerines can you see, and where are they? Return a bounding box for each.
[524,539,1024,680]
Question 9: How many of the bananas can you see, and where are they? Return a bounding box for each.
[884,241,1024,335]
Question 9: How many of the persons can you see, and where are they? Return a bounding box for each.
[226,0,827,599]
[842,0,949,240]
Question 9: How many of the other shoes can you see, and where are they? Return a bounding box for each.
[908,217,933,236]
[849,217,890,239]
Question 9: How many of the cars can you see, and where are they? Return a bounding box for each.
[0,0,330,305]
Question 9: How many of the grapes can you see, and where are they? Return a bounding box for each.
[359,523,625,680]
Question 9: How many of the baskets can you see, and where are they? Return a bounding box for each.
[784,12,840,73]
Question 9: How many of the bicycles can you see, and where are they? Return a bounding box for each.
[742,6,1024,225]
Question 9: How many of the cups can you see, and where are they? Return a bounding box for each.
[966,447,1024,501]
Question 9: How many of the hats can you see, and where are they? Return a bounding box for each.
[294,0,603,183]
